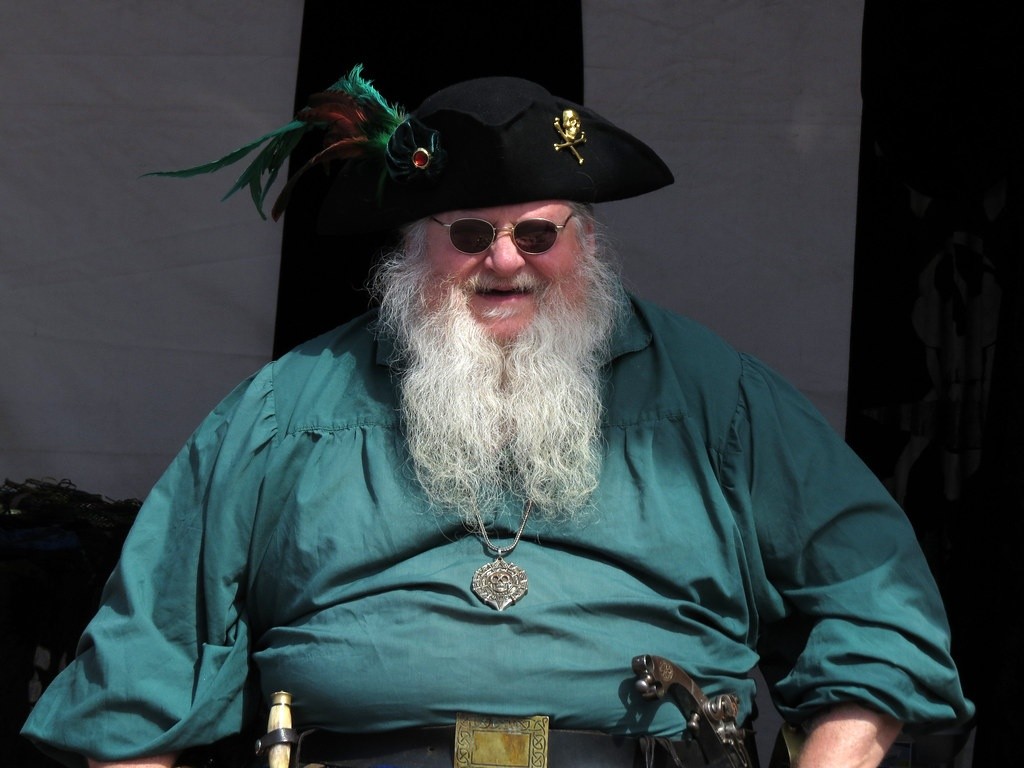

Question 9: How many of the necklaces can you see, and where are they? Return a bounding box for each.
[471,502,534,616]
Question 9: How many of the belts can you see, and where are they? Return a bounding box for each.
[238,716,707,768]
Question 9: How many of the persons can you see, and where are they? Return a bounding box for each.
[18,63,975,768]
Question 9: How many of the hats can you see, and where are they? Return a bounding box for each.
[136,64,675,223]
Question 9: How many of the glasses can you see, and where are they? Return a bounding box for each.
[428,209,576,255]
